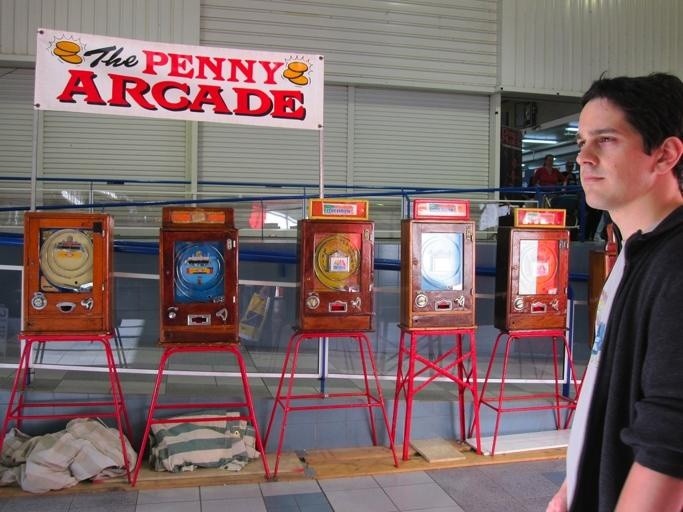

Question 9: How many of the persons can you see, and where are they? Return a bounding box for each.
[546,73,682,512]
[528,154,567,191]
[562,160,577,184]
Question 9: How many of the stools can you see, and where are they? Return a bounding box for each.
[0,332,134,487]
[389,326,482,461]
[262,330,398,479]
[134,339,272,483]
[470,327,580,457]
